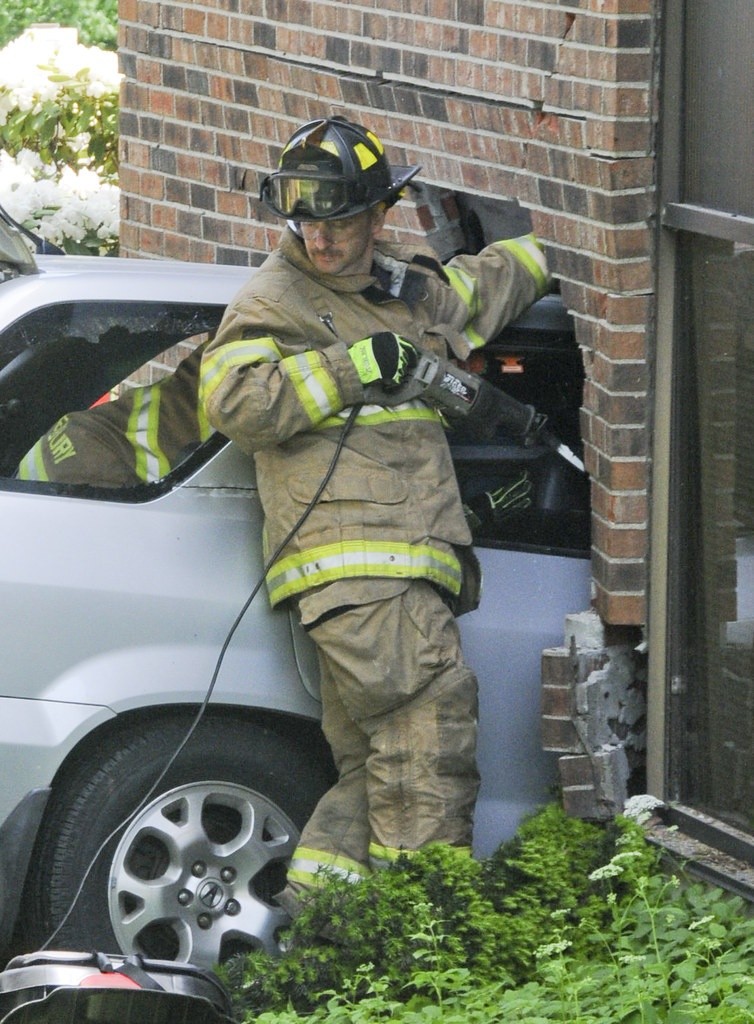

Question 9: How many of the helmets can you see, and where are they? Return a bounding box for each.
[271,115,423,205]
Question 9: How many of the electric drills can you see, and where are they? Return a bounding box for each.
[360,336,585,475]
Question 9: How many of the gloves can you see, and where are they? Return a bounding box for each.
[347,330,420,394]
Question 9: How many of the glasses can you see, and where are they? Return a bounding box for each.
[264,161,391,220]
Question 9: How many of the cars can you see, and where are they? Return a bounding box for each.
[0,254,753,975]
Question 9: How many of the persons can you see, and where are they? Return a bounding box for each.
[19,340,208,490]
[201,114,559,941]
